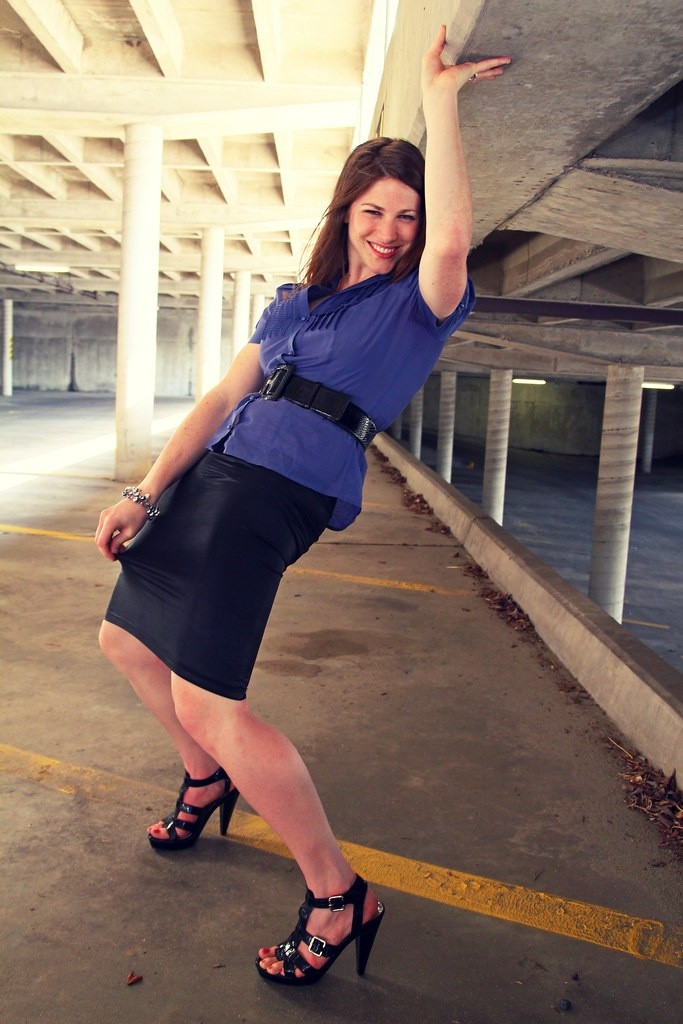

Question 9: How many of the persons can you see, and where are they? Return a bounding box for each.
[91,23,513,986]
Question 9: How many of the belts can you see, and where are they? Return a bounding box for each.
[262,363,378,452]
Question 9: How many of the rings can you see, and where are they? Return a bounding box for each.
[469,74,477,81]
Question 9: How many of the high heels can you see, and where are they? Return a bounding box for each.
[148,766,241,852]
[255,872,386,988]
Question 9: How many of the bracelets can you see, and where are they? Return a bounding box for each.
[122,486,161,522]
